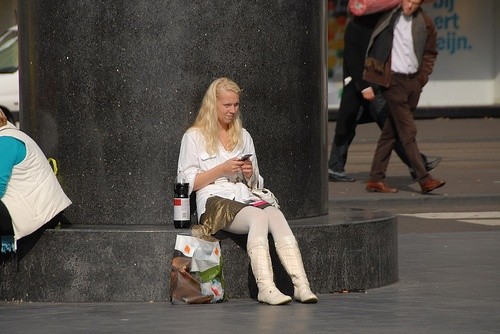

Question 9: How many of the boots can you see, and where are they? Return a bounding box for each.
[274,235,319,303]
[246,238,293,305]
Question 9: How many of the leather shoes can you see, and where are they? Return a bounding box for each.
[366,179,398,194]
[410,157,445,179]
[421,176,447,195]
[328,172,356,183]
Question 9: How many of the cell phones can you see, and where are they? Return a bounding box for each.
[237,153,252,162]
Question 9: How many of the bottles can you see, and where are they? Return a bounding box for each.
[173,168,191,229]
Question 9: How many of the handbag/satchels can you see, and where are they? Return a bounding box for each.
[168,233,226,305]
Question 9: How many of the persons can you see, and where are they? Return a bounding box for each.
[177,78,319,304]
[328,0,442,182]
[363,0,445,193]
[0,107,72,263]
